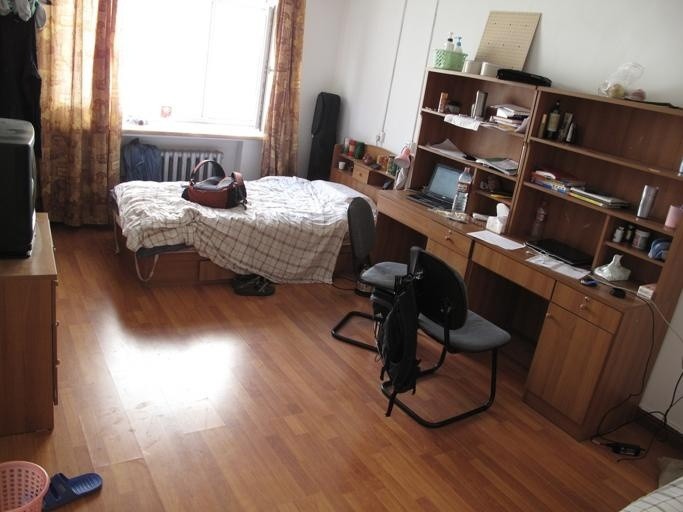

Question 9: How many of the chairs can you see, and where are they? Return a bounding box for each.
[331,197,408,353]
[379,246,511,428]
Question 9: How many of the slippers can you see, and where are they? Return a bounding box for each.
[236,282,275,297]
[38,472,102,509]
[231,272,270,287]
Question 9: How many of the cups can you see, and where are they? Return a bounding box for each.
[665,205,683,229]
[637,185,658,219]
[339,162,345,170]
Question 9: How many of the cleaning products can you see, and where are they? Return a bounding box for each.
[446,32,454,52]
[544,101,561,140]
[455,37,462,53]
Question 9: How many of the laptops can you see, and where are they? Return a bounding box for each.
[405,163,464,212]
[525,237,593,266]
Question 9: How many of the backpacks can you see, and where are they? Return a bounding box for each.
[182,160,248,209]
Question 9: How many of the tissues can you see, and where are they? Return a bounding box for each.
[485,204,509,235]
[594,254,631,281]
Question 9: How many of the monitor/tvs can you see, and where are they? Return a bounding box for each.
[0,114,42,261]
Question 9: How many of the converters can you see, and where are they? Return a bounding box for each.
[609,441,642,458]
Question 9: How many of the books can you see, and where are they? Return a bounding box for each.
[526,167,587,211]
[566,186,630,210]
[473,155,520,179]
[489,102,530,135]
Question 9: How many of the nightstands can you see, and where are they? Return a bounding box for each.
[329,142,396,205]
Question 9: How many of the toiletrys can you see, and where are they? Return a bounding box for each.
[558,112,572,142]
[566,123,574,145]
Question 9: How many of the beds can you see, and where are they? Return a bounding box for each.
[112,175,377,284]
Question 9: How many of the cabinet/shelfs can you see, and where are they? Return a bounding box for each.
[0,213,60,438]
[376,68,539,319]
[469,86,683,441]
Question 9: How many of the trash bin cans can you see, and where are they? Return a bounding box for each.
[0,461,50,512]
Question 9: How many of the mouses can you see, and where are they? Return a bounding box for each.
[580,276,598,288]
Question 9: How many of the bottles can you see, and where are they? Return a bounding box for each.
[546,100,562,140]
[344,137,350,152]
[349,140,357,155]
[612,226,624,242]
[387,155,394,170]
[451,167,472,212]
[530,200,548,238]
[354,141,365,159]
[631,229,650,250]
